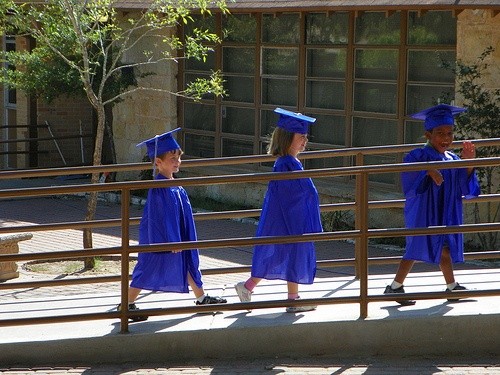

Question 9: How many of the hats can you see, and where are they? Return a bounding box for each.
[134,128,184,160]
[274,107,316,133]
[411,104,467,130]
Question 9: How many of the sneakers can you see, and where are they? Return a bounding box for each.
[286,296,316,312]
[233,282,253,312]
[117,304,148,322]
[196,296,227,315]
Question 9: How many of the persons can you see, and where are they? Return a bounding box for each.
[235,108,324,313]
[383,104,481,306]
[118,127,226,321]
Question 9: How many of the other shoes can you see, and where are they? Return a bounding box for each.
[444,282,469,303]
[383,286,415,306]
